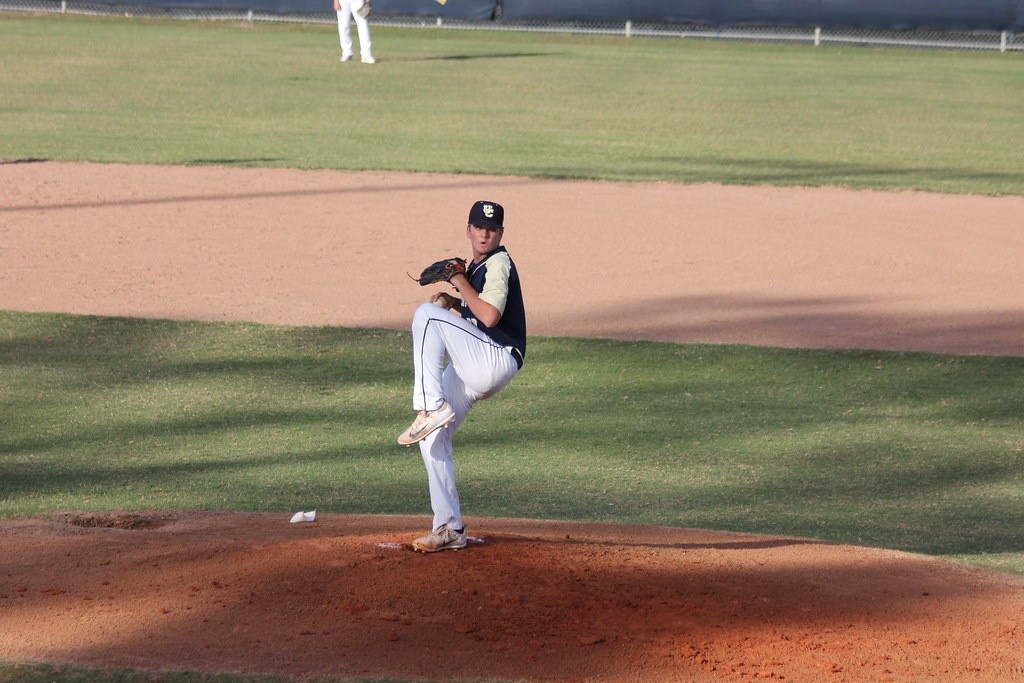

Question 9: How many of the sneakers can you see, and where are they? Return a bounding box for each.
[361,56,375,64]
[412,523,468,552]
[397,401,456,448]
[340,55,352,62]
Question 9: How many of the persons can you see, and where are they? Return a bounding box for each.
[333,0,376,64]
[398,201,526,552]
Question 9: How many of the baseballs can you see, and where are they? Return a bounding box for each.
[432,296,449,309]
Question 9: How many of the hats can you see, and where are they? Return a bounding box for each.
[468,201,504,229]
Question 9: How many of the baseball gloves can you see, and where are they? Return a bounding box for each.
[357,2,372,19]
[419,257,466,287]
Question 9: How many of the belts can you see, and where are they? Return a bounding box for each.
[510,347,523,370]
